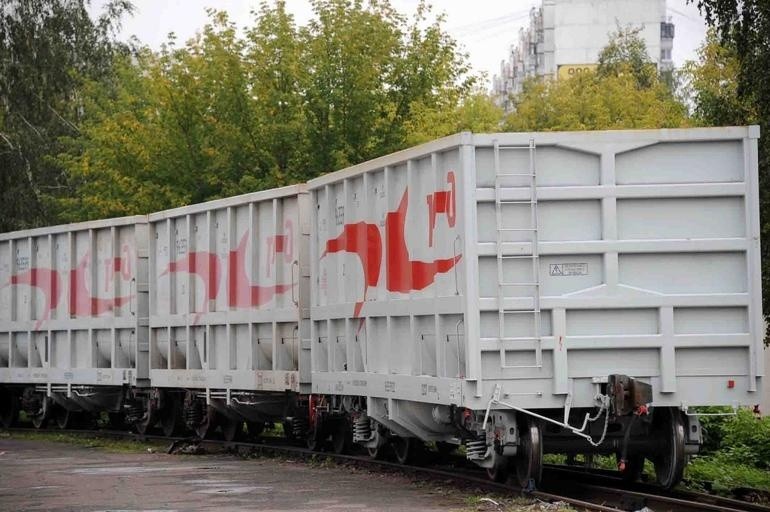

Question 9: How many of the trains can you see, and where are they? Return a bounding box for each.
[1,126,765,491]
